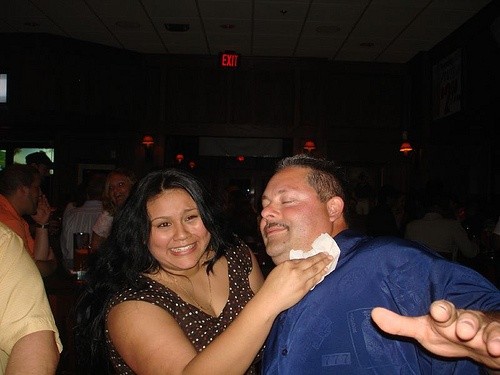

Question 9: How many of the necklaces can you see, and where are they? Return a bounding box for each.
[166,268,214,315]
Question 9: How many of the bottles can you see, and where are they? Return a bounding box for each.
[488,233,497,264]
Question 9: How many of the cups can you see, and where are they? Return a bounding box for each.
[72,232,90,272]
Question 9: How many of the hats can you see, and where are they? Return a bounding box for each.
[25,151,55,170]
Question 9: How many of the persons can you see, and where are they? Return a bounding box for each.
[91,166,136,249]
[0,219,65,375]
[366,182,407,235]
[404,198,480,262]
[1,163,56,261]
[259,154,500,375]
[28,150,161,199]
[70,169,332,374]
